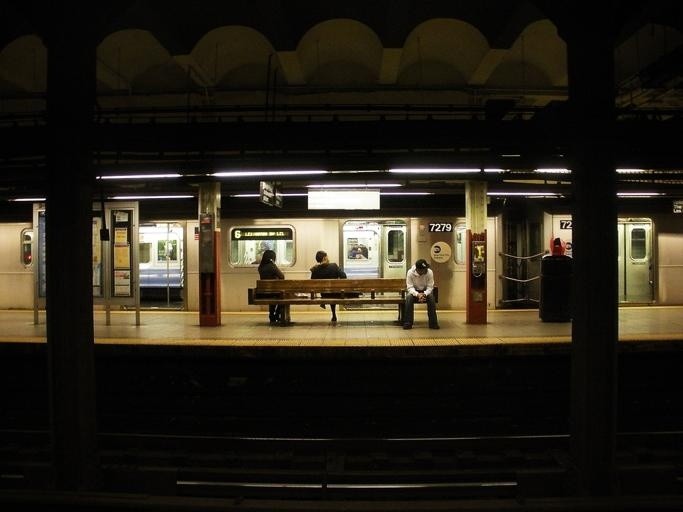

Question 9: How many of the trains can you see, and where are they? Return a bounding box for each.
[0,189,683,315]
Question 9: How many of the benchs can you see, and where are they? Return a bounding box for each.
[247,279,437,327]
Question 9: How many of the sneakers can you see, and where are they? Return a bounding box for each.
[403,322,412,329]
[320,303,325,309]
[429,323,440,329]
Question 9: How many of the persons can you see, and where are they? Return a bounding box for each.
[400,259,440,331]
[350,243,368,260]
[309,251,346,321]
[549,236,565,256]
[258,249,285,327]
[248,241,274,264]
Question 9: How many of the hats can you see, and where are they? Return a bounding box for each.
[415,259,429,270]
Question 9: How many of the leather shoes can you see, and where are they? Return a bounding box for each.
[269,314,275,322]
[274,315,281,321]
